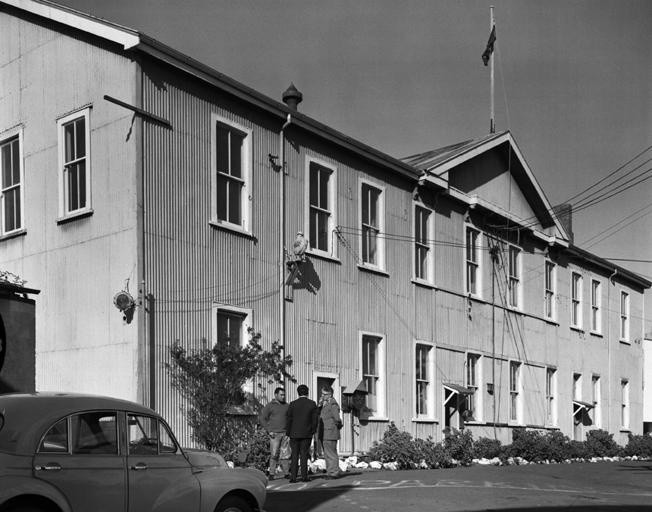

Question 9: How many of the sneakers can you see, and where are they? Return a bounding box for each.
[324,476,336,480]
[285,474,290,478]
[269,475,276,480]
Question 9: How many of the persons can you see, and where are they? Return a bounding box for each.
[285,383,318,483]
[317,385,343,479]
[286,232,308,272]
[259,387,291,480]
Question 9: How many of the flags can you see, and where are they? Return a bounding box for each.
[481,25,497,66]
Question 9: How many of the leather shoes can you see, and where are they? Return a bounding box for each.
[290,480,296,483]
[303,478,312,482]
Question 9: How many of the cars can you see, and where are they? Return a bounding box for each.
[0,389,269,512]
